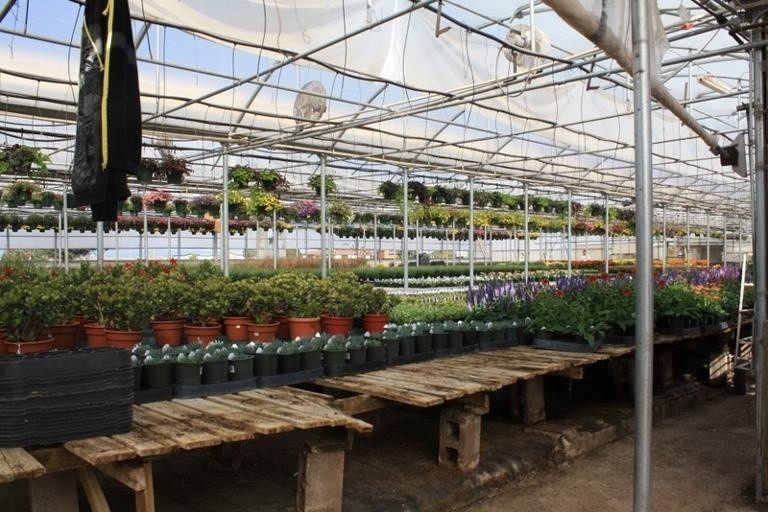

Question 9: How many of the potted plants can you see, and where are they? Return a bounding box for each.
[0,251,141,341]
